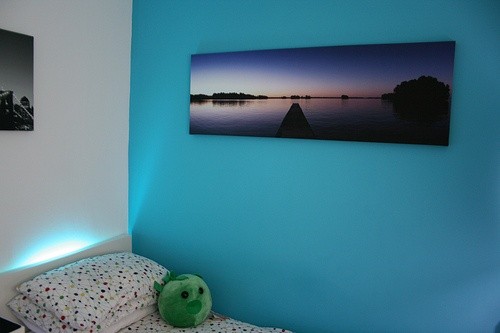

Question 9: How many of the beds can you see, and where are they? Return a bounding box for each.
[0,234,293,333]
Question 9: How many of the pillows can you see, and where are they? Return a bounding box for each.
[6,252,172,333]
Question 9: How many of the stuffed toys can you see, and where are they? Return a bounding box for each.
[154,269,212,328]
